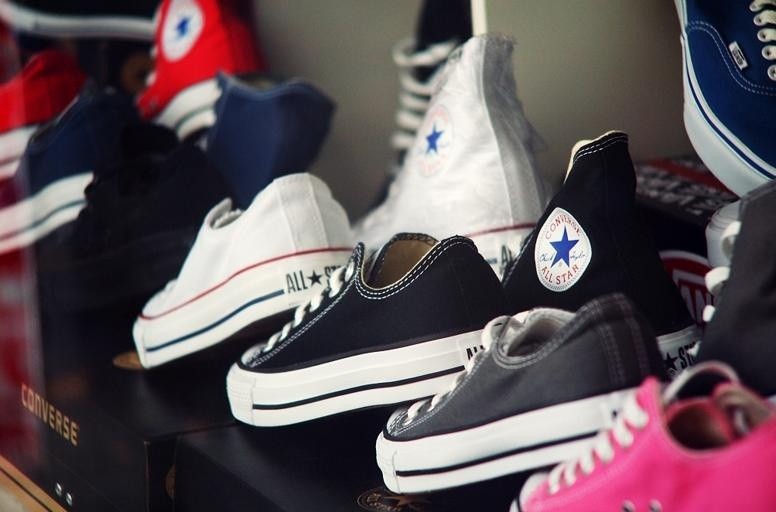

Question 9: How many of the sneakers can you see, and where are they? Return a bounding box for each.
[376,292,652,493]
[497,135,700,371]
[227,231,514,427]
[521,362,774,512]
[133,174,357,370]
[350,2,553,283]
[676,2,774,195]
[686,180,774,400]
[0,0,333,345]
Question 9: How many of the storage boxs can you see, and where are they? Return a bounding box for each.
[560,154,741,331]
